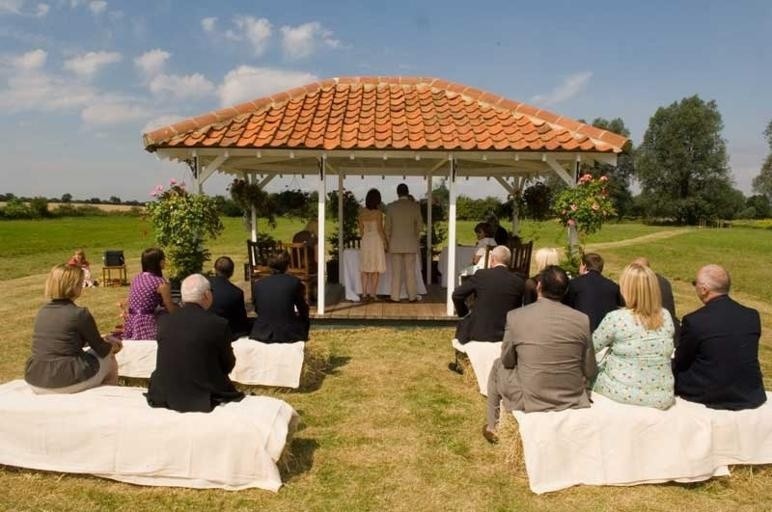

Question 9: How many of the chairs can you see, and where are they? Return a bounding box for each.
[102,251,127,287]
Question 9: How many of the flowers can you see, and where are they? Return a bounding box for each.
[141,179,226,290]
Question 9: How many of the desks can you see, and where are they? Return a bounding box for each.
[438,245,477,289]
[344,247,427,303]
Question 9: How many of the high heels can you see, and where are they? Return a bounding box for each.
[361,296,369,303]
[369,296,383,303]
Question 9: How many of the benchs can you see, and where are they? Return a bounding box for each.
[461,240,533,303]
[82,334,306,391]
[246,239,318,305]
[451,337,677,395]
[0,379,299,494]
[495,389,772,494]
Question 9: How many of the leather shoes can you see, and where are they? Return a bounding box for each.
[408,298,419,303]
[385,298,401,304]
[482,422,499,446]
[448,360,465,376]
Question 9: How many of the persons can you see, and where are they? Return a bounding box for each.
[248,251,311,343]
[448,223,767,445]
[293,215,318,306]
[142,274,245,413]
[208,257,249,342]
[68,250,94,288]
[117,248,173,340]
[24,263,123,395]
[358,188,386,301]
[383,183,423,301]
